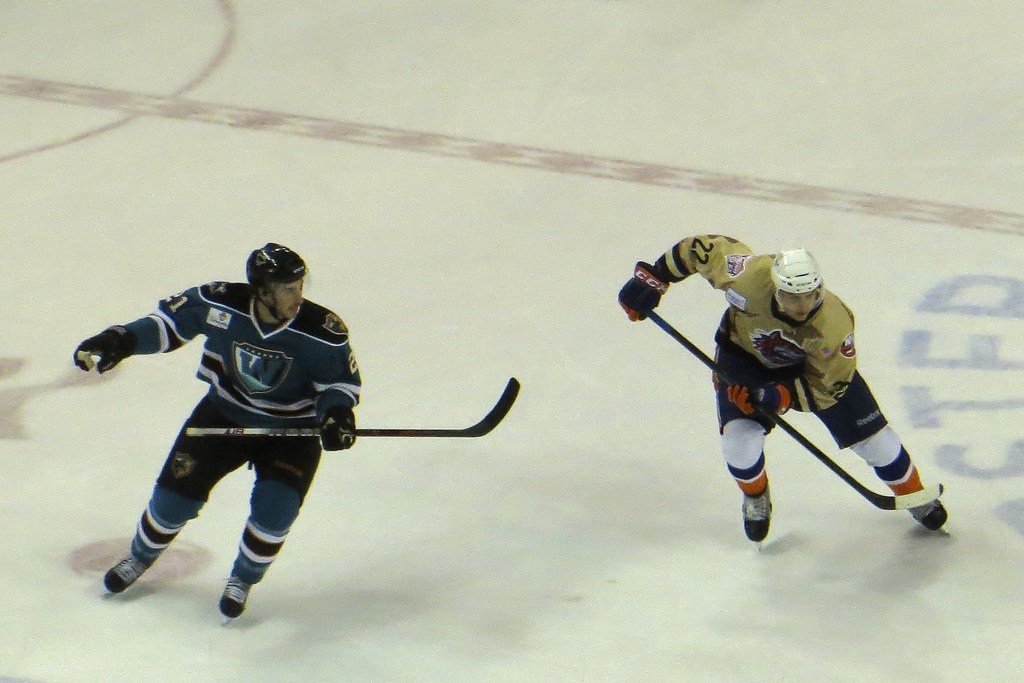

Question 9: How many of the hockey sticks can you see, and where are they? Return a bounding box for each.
[645,309,946,511]
[184,376,521,440]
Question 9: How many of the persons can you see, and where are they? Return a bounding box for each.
[619,233,949,542]
[74,243,363,620]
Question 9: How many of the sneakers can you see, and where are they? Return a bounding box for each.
[218,574,252,618]
[743,483,771,551]
[907,499,952,540]
[105,556,148,594]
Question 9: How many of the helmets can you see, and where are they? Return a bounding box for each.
[770,249,824,294]
[245,242,306,297]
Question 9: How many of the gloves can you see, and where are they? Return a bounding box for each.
[74,324,134,373]
[727,382,790,415]
[618,261,669,321]
[319,405,357,451]
